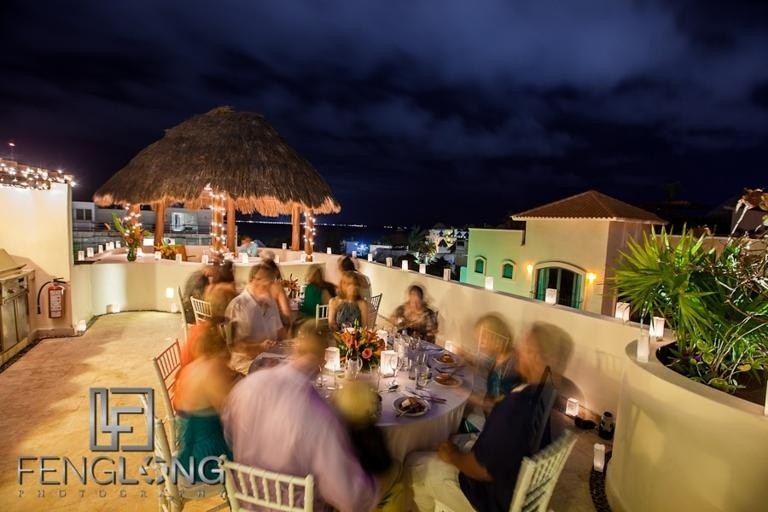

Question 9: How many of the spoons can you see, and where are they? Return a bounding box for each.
[377,384,399,393]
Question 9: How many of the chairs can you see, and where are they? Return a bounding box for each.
[140,270,582,512]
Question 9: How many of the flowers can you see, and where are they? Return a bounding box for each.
[110,210,153,249]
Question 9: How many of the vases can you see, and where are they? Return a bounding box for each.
[127,249,136,261]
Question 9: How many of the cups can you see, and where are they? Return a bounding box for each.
[291,285,306,303]
[415,366,429,390]
[313,346,380,401]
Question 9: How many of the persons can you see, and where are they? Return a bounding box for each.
[404,320,585,512]
[219,320,403,511]
[173,237,523,481]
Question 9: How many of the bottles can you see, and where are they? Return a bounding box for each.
[372,326,395,351]
[389,326,430,381]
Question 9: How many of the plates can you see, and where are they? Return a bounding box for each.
[432,350,464,388]
[392,396,428,416]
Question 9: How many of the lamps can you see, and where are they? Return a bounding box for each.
[106,304,113,313]
[649,314,667,341]
[72,240,122,261]
[593,443,606,473]
[79,319,87,333]
[615,302,631,322]
[635,335,649,363]
[323,246,426,274]
[152,242,309,266]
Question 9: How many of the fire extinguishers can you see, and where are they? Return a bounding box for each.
[37,278,67,319]
